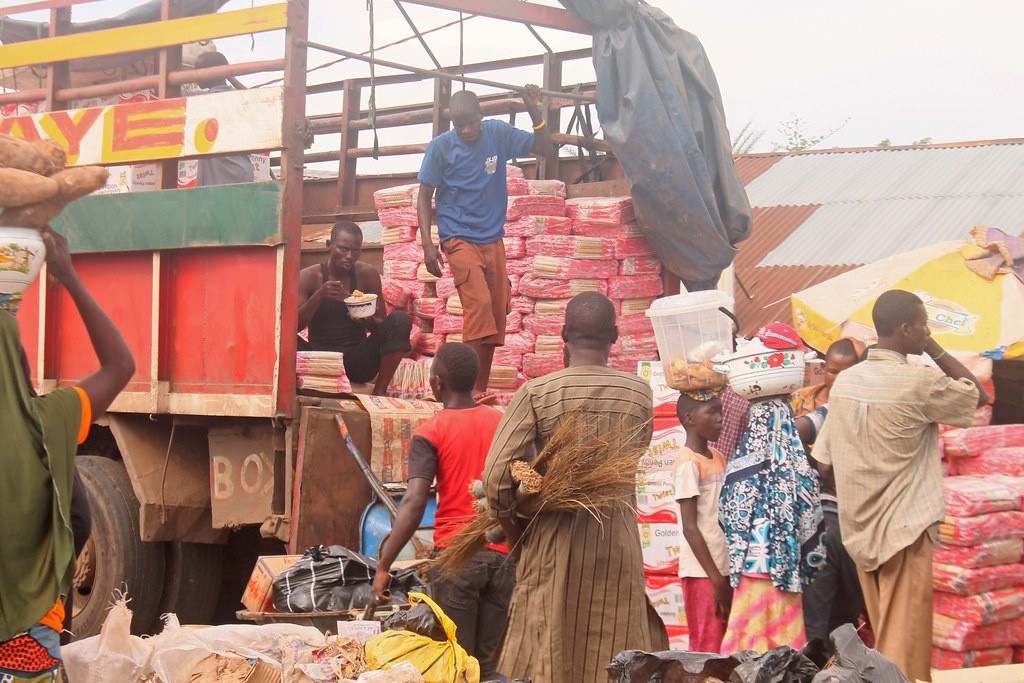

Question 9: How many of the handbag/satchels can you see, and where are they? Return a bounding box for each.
[362,591,480,683]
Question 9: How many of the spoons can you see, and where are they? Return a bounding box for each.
[342,288,362,297]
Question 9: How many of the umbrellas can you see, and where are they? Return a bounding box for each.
[763,227,1024,369]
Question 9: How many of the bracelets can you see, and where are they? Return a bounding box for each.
[934,351,945,360]
[533,121,546,130]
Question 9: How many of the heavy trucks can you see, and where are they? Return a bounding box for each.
[0,0,752,646]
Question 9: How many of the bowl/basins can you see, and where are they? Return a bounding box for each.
[344,294,378,318]
[711,349,818,400]
[0,225,46,294]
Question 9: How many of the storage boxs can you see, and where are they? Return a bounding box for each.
[240,555,304,612]
[645,290,735,391]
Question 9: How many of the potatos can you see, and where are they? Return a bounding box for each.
[0,132,111,229]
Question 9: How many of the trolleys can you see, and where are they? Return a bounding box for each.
[236,537,435,637]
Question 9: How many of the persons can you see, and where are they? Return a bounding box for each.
[372,341,517,683]
[300,221,413,396]
[794,338,867,645]
[194,52,254,186]
[417,84,555,404]
[482,290,654,683]
[673,394,730,653]
[0,226,136,683]
[718,399,827,655]
[813,289,991,683]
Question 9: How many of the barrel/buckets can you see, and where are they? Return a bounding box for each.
[645,289,735,390]
